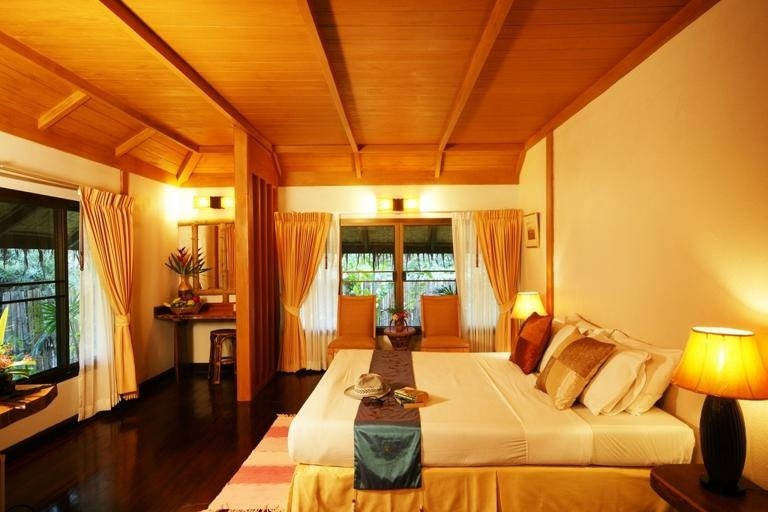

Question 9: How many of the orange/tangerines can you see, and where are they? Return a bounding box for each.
[171,302,184,308]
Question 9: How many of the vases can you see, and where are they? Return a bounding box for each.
[0,374,16,401]
[395,321,404,333]
[176,275,193,300]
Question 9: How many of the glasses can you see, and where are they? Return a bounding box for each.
[362,395,387,407]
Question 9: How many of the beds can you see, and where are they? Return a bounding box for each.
[278,313,693,511]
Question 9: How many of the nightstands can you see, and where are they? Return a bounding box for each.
[643,461,767,512]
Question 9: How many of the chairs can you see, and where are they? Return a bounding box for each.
[327,293,376,372]
[416,292,470,353]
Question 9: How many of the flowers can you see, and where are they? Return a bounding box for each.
[384,302,410,333]
[164,244,207,277]
[0,301,33,379]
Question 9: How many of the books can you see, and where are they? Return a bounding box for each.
[394,387,428,408]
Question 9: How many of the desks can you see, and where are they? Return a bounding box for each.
[0,383,57,511]
[383,325,415,351]
[153,302,237,382]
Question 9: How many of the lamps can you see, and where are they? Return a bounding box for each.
[668,324,767,496]
[390,197,406,213]
[205,195,225,210]
[511,288,547,322]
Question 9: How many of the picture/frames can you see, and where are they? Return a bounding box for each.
[522,211,539,249]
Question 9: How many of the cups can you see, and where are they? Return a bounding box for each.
[233,303,236,318]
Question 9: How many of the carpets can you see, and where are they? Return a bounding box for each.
[202,408,292,511]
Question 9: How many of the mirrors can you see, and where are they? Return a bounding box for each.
[177,219,236,296]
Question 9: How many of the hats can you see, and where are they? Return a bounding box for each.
[344,373,392,399]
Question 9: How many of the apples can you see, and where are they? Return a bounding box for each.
[192,296,200,303]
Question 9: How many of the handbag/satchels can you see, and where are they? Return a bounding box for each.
[394,386,429,403]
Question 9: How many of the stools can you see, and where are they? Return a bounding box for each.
[205,326,235,386]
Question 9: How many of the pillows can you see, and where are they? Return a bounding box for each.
[536,315,586,377]
[561,311,600,336]
[509,310,553,376]
[611,330,682,415]
[587,328,650,416]
[536,325,617,412]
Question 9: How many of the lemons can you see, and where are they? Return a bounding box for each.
[173,298,182,304]
[186,300,195,306]
